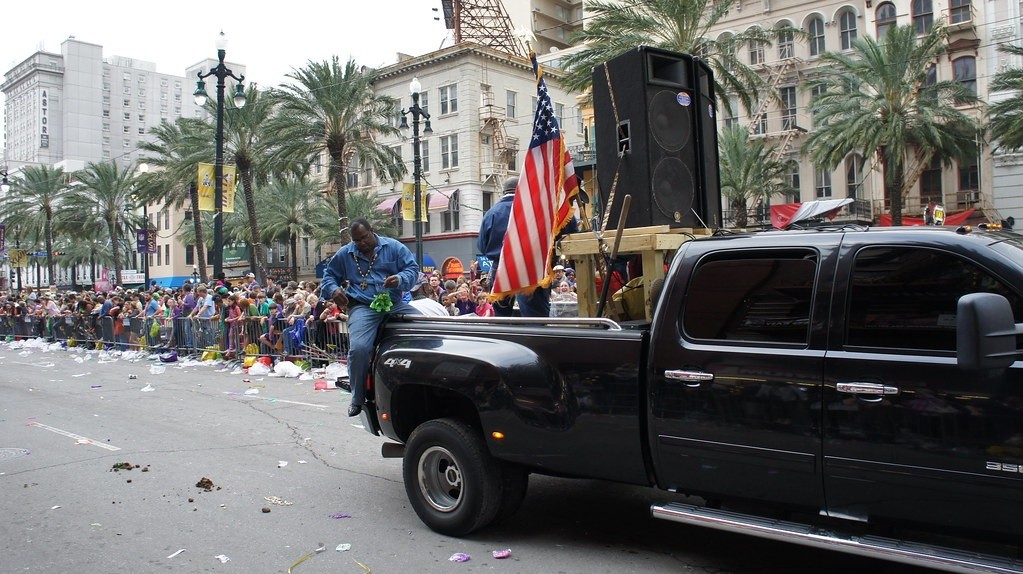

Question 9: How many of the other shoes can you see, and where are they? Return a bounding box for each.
[348,403,362,417]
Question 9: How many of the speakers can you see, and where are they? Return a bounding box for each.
[591,44,724,229]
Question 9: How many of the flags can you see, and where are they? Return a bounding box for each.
[491,52,579,296]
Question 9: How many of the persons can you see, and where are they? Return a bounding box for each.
[476,176,579,317]
[0,264,577,369]
[321,216,425,417]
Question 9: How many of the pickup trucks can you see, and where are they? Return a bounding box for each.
[358,225,1023,574]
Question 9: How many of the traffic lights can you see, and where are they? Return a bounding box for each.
[10,271,15,283]
[55,251,66,256]
[26,252,34,255]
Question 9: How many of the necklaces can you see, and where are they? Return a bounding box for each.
[354,254,378,290]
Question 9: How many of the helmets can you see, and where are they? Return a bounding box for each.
[503,177,518,195]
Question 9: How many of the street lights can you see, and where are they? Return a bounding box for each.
[190,26,248,282]
[0,164,22,291]
[397,75,433,272]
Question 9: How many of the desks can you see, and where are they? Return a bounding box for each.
[556,225,760,323]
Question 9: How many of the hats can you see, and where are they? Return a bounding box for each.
[115,286,122,292]
[297,281,305,289]
[29,292,36,299]
[244,272,255,278]
[553,265,567,272]
[165,288,172,294]
[268,304,277,309]
[43,292,51,298]
